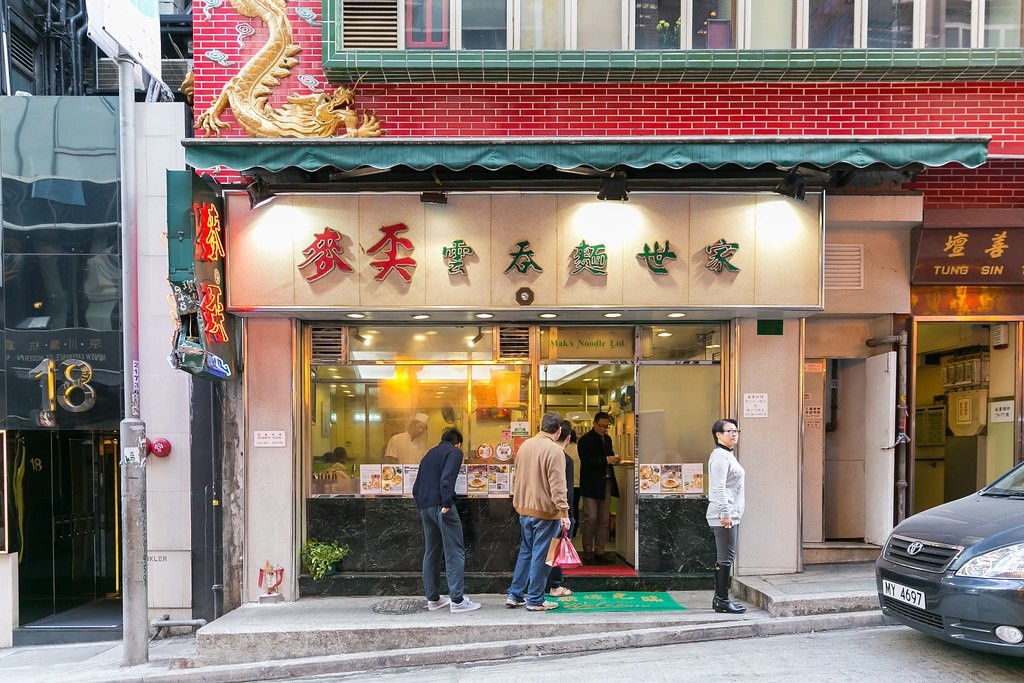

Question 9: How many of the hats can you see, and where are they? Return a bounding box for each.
[416,413,429,425]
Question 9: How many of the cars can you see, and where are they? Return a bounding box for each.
[875,460,1024,660]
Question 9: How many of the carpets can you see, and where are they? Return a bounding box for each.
[543,592,688,613]
[561,566,637,576]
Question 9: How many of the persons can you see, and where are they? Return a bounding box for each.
[550,420,574,597]
[577,411,620,562]
[322,446,352,463]
[565,430,581,537]
[505,411,571,611]
[381,431,399,459]
[384,413,429,464]
[705,419,746,614]
[413,427,481,613]
[606,456,609,464]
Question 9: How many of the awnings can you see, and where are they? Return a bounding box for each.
[182,134,995,192]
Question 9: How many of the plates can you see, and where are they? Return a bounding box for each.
[467,465,516,493]
[640,464,703,493]
[361,465,402,493]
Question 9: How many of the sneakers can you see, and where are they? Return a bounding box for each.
[428,597,451,610]
[550,586,573,596]
[506,597,526,605]
[526,600,558,610]
[450,596,481,612]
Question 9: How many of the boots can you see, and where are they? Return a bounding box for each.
[715,563,746,614]
[713,563,743,609]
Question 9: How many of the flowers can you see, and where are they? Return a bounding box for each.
[656,17,681,45]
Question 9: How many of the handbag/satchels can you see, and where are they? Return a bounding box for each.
[545,525,564,566]
[552,529,583,568]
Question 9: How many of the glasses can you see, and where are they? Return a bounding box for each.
[718,429,740,435]
[595,420,610,429]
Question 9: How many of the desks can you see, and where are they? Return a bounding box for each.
[706,19,730,49]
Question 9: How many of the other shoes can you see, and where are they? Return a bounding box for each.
[582,553,592,560]
[594,554,612,562]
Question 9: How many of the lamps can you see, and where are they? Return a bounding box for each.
[597,170,631,202]
[419,173,448,204]
[246,173,276,210]
[774,166,807,201]
